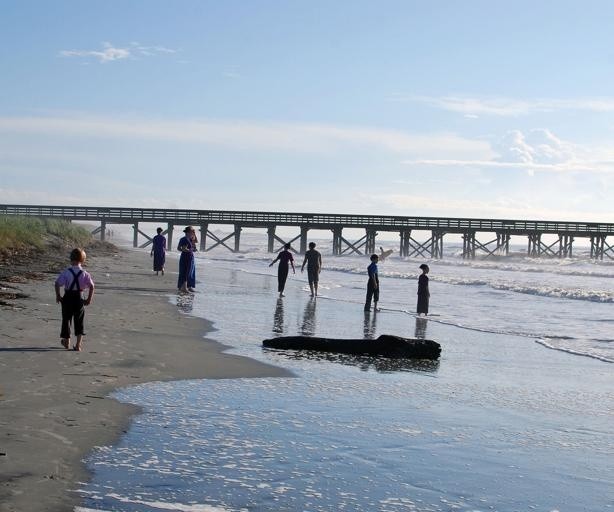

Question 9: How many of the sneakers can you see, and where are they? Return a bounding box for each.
[73,344,80,350]
[60,338,71,349]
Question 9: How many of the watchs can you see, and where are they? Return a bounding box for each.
[363,254,381,313]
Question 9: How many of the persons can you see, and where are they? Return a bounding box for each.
[380,247,385,262]
[272,295,284,334]
[416,264,431,316]
[187,230,198,291]
[150,227,167,276]
[301,296,319,336]
[268,243,296,297]
[364,310,377,340]
[54,247,94,353]
[301,242,322,297]
[177,292,195,315]
[177,225,193,294]
[415,316,428,340]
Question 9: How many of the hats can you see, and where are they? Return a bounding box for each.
[183,227,194,232]
